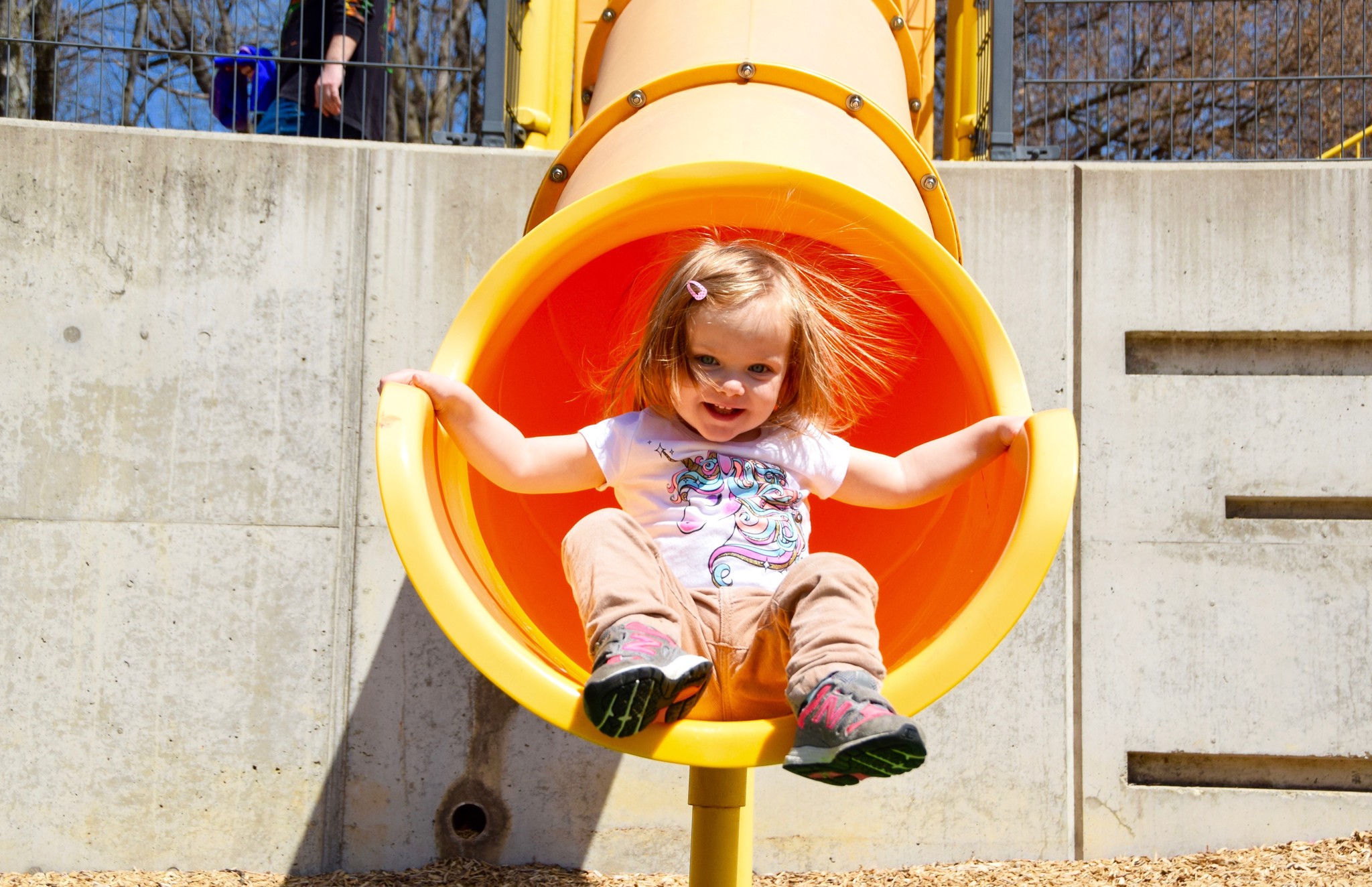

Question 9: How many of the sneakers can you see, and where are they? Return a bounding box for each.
[583,621,713,738]
[783,689,927,786]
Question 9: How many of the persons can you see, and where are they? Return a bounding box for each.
[226,0,388,142]
[377,232,1031,787]
